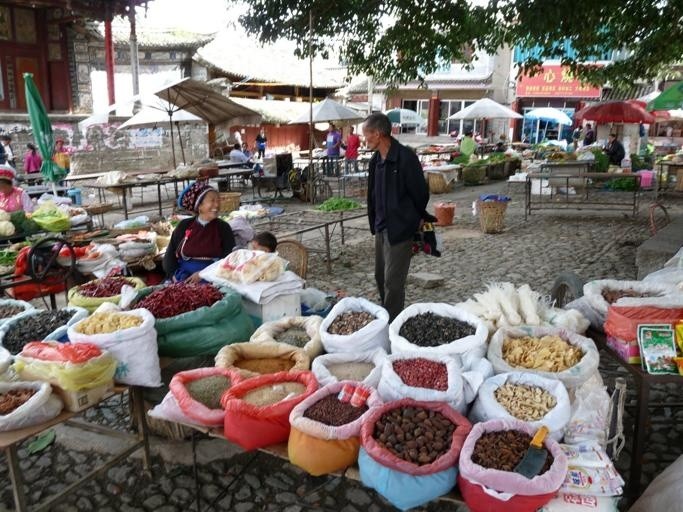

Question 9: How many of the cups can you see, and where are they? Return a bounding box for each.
[338,384,353,404]
[350,382,368,409]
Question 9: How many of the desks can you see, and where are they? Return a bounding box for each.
[524,173,642,222]
[15,144,457,214]
[539,157,594,201]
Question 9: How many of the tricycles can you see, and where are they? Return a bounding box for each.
[252,158,333,200]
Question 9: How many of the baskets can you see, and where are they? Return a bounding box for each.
[219,192,241,213]
[157,354,216,397]
[128,393,198,439]
[427,172,450,194]
[109,227,150,236]
[82,204,112,215]
[478,199,507,234]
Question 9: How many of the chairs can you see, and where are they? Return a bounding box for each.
[646,202,670,237]
[275,239,308,279]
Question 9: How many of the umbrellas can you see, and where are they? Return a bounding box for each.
[444,96,524,161]
[22,70,65,197]
[522,104,574,145]
[285,97,367,126]
[570,77,682,130]
[116,101,202,185]
[381,106,430,127]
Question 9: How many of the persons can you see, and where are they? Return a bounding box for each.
[459,128,479,159]
[569,122,625,165]
[250,230,278,254]
[324,121,341,175]
[161,180,236,284]
[227,120,270,185]
[51,137,70,193]
[344,125,361,176]
[21,143,41,187]
[0,166,31,214]
[492,134,508,153]
[0,133,15,168]
[361,112,430,325]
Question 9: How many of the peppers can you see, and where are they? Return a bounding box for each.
[131,281,227,318]
[79,276,136,297]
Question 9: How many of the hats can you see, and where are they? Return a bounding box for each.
[0,166,16,181]
[177,178,214,211]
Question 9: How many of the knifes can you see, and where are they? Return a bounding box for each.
[513,425,548,479]
